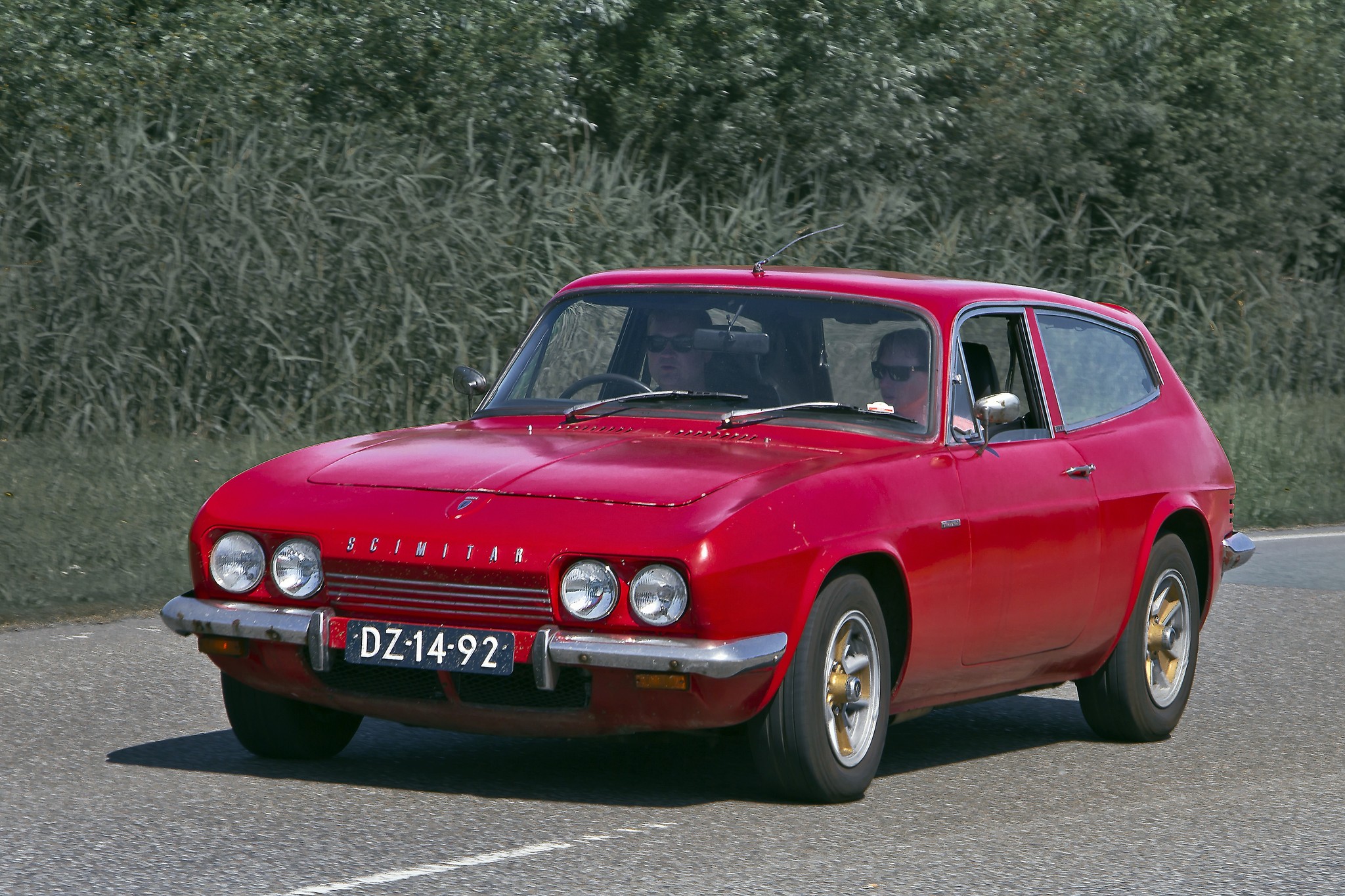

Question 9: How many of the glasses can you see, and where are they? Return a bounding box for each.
[871,362,929,381]
[646,335,694,353]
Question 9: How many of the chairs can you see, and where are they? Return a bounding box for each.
[951,339,1025,442]
[698,322,784,413]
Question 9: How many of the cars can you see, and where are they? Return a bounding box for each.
[158,266,1259,806]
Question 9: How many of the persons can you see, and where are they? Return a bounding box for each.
[869,329,984,438]
[634,303,782,410]
